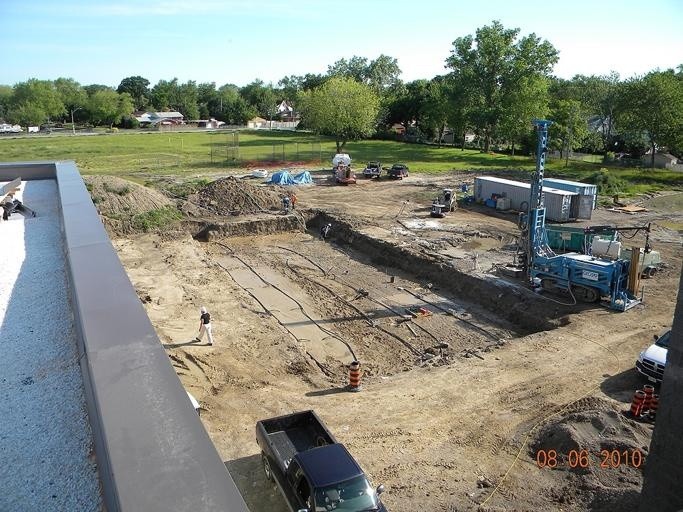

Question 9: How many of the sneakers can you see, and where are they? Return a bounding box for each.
[208,343,212,346]
[196,337,201,342]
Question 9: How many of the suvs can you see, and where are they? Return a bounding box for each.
[633,328,675,386]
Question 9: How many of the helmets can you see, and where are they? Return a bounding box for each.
[201,307,206,311]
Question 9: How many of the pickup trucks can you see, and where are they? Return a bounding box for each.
[252,406,389,511]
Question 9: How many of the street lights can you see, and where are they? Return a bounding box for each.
[70,106,81,135]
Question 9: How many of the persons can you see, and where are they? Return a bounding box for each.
[290,194,296,209]
[318,223,331,240]
[431,197,440,211]
[195,306,212,346]
[281,196,289,212]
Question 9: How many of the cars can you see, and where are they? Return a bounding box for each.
[331,152,409,185]
[0,122,63,133]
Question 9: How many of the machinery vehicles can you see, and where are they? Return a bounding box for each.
[505,119,650,314]
[430,188,458,213]
